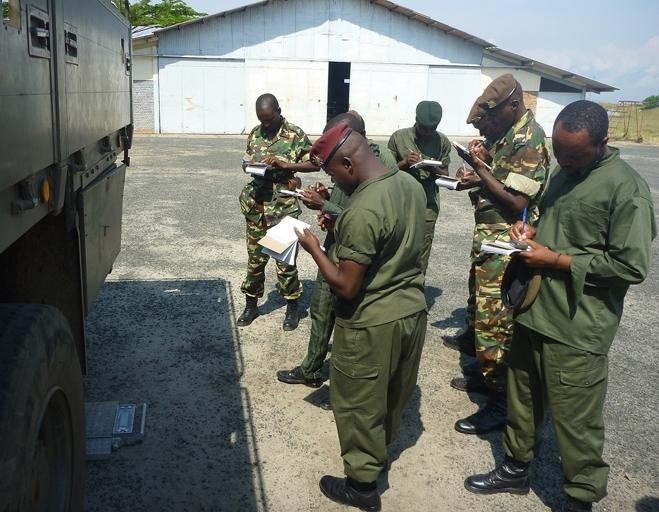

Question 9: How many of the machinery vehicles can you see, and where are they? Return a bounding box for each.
[0,1,135,510]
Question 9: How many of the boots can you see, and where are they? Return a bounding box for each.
[283,299,298,330]
[551,490,592,512]
[277,365,323,387]
[450,377,489,394]
[454,389,506,434]
[319,474,381,511]
[321,399,332,410]
[464,451,531,495]
[461,358,482,376]
[237,294,259,326]
[443,325,475,357]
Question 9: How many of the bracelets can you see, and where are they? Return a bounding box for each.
[553,250,563,269]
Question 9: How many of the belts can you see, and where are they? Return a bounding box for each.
[474,211,531,224]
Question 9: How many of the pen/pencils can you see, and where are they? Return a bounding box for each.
[309,186,334,192]
[407,149,419,157]
[520,208,528,239]
[473,139,488,149]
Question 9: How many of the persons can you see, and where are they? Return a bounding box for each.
[451,74,551,434]
[388,101,452,290]
[443,97,500,375]
[276,114,406,411]
[293,123,428,509]
[464,101,657,511]
[237,93,320,332]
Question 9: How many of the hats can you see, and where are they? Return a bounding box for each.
[309,121,353,169]
[478,74,517,112]
[501,255,542,311]
[467,96,488,127]
[415,101,442,129]
